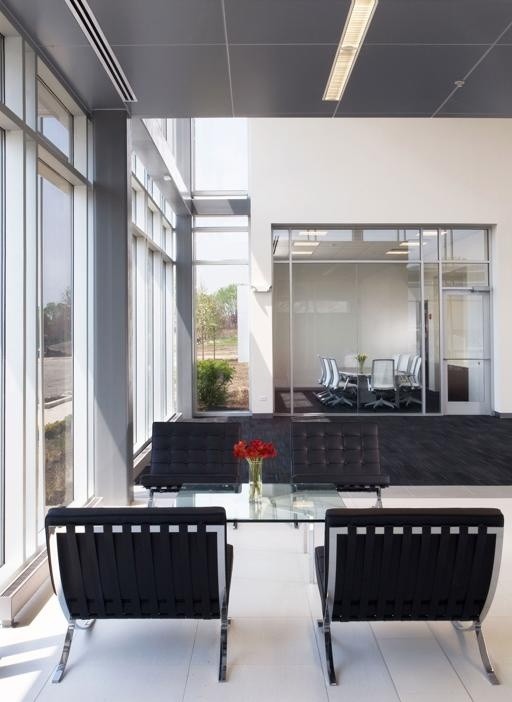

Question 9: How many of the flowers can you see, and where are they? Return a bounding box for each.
[233,438,277,504]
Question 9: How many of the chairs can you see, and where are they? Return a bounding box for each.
[140,421,240,506]
[314,508,505,686]
[313,353,424,409]
[43,506,234,684]
[289,422,390,527]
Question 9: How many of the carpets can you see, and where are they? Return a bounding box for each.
[134,416,512,485]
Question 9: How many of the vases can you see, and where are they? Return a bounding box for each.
[248,460,262,504]
